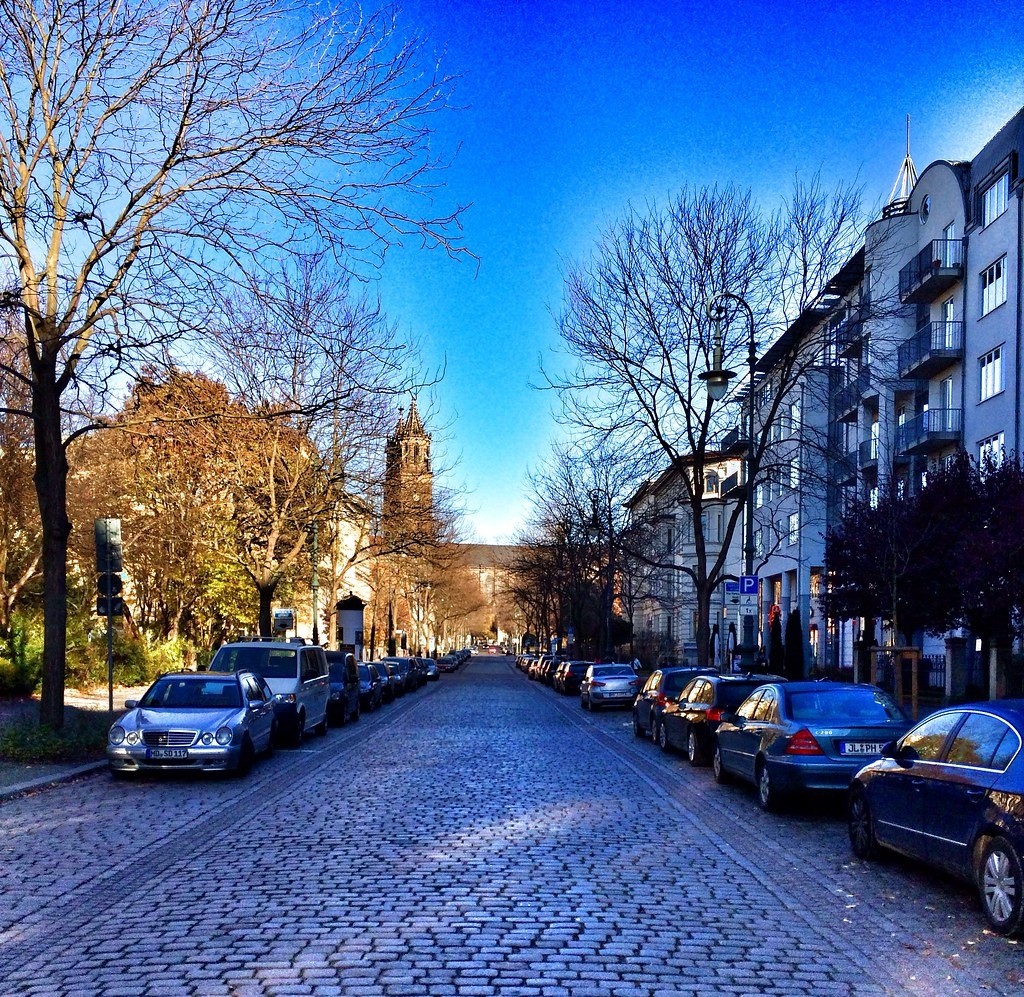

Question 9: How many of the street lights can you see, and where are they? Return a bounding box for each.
[558,549,575,659]
[588,488,615,664]
[695,291,758,671]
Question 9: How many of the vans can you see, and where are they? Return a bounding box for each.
[197,642,330,750]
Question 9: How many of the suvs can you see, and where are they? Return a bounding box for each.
[322,650,360,729]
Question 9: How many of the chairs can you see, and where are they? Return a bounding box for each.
[168,687,203,706]
[271,657,294,677]
[223,684,239,705]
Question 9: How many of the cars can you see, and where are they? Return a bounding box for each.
[380,645,479,694]
[714,682,918,816]
[631,665,719,746]
[356,663,381,710]
[482,644,490,649]
[488,646,496,653]
[660,672,788,769]
[499,642,509,653]
[580,664,641,712]
[850,700,1024,938]
[372,661,395,703]
[386,661,404,701]
[106,668,279,783]
[516,652,594,695]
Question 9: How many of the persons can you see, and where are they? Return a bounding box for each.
[417,649,422,657]
[426,650,429,658]
[343,653,360,723]
[754,647,768,676]
[409,648,413,656]
[433,650,437,660]
[448,647,455,655]
[630,656,642,676]
